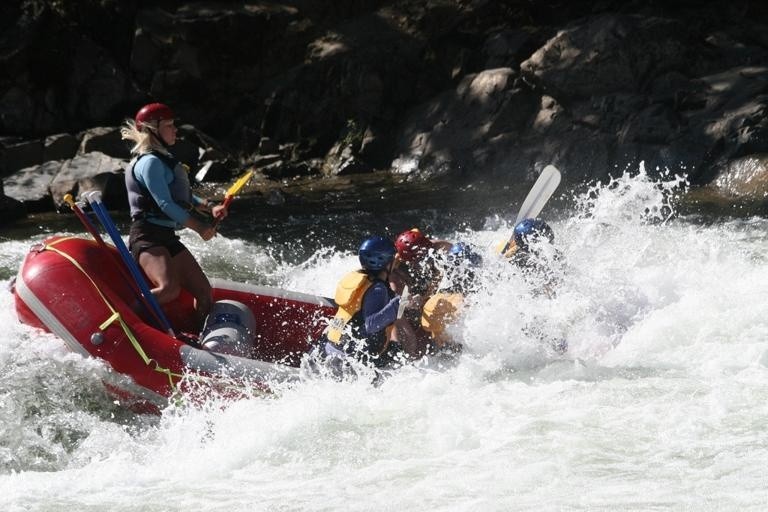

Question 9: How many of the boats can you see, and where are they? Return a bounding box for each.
[57,216,497,424]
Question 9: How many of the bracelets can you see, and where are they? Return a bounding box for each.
[205,201,216,213]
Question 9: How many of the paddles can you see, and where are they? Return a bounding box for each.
[213,169,256,229]
[396,285,420,321]
[497,162,562,256]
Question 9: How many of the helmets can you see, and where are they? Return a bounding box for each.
[447,242,482,264]
[514,218,554,248]
[359,237,397,270]
[136,104,174,131]
[395,232,433,262]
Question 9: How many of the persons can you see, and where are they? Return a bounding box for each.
[120,103,228,327]
[507,218,555,271]
[322,228,481,369]
